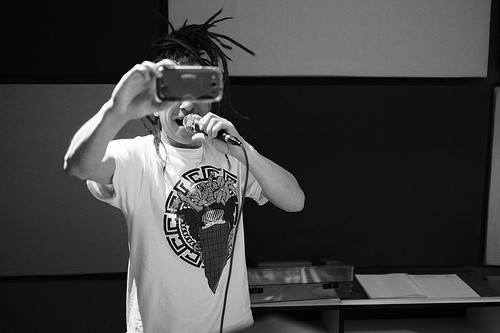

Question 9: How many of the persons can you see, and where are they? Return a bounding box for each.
[64,6,305,333]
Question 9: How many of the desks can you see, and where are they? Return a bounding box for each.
[246,264,500,333]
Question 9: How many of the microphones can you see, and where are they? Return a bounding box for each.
[183,114,242,147]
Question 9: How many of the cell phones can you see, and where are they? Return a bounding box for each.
[156,65,223,103]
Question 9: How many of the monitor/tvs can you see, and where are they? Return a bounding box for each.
[0,0,169,84]
[209,75,496,275]
